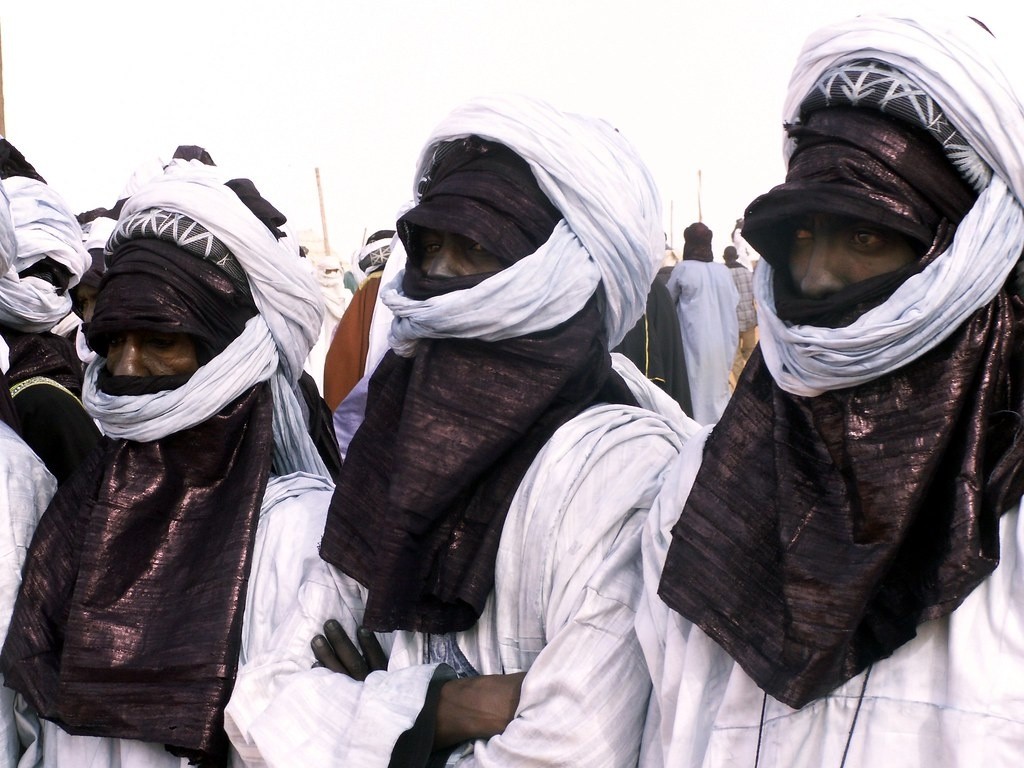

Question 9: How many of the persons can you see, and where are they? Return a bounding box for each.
[1,131,396,768]
[224,101,682,768]
[609,218,760,427]
[634,16,1023,768]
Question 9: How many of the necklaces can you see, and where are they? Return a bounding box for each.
[755,666,872,768]
